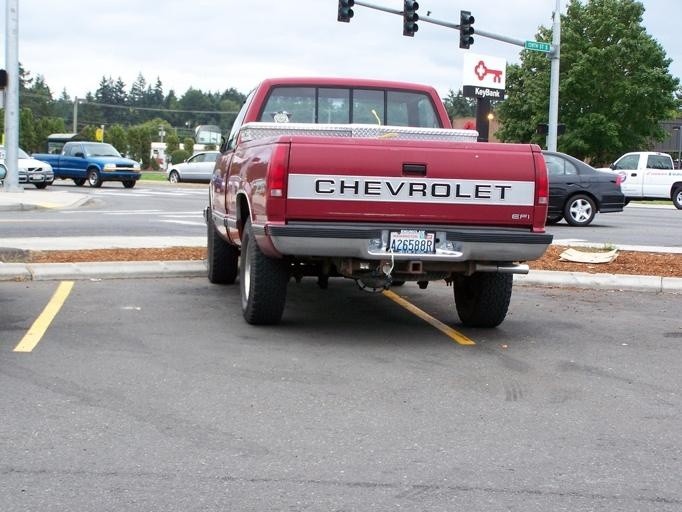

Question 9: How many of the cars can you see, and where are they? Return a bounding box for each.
[540,149,626,227]
[0,145,54,189]
[166,150,222,184]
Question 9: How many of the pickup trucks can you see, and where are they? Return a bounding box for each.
[596,150,682,211]
[202,75,554,333]
[31,141,142,188]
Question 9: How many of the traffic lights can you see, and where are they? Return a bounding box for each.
[338,0,355,23]
[459,10,475,50]
[403,0,419,37]
